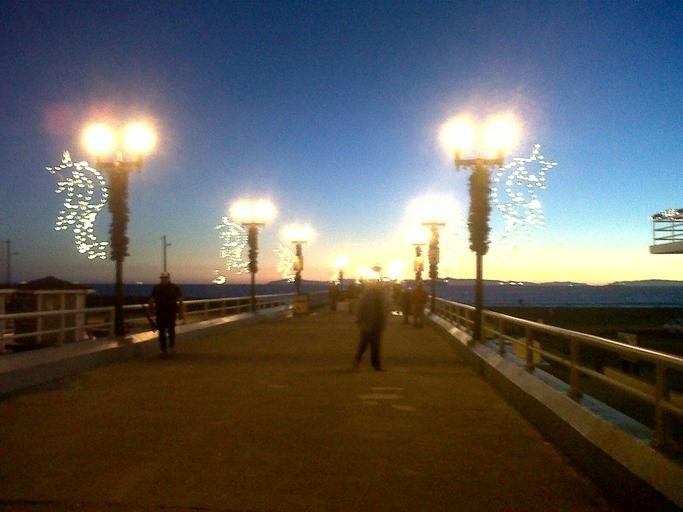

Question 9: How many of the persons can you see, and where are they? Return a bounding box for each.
[355,288,384,370]
[327,280,427,329]
[148,271,182,360]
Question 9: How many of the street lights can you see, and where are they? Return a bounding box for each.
[399,189,455,312]
[330,255,348,291]
[435,109,521,342]
[227,195,277,312]
[282,222,312,293]
[80,118,161,334]
